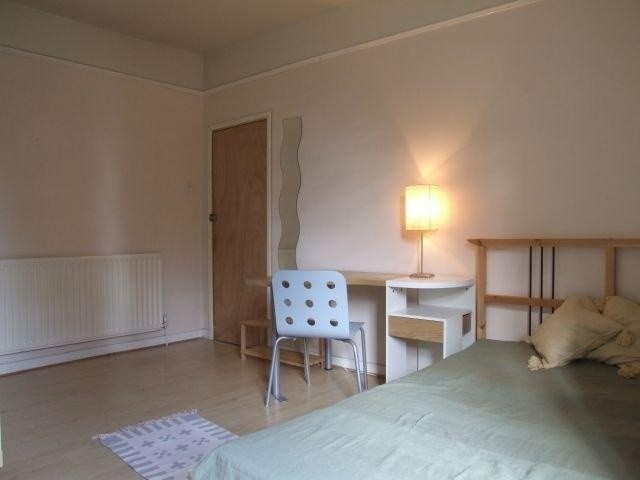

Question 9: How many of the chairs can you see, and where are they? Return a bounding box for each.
[264,269,368,408]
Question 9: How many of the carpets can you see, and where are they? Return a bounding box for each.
[92,409,239,480]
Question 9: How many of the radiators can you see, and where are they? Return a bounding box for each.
[0,251,165,357]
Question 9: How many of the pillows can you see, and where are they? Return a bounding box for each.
[519,292,640,380]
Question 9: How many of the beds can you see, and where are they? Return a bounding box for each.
[193,238,640,480]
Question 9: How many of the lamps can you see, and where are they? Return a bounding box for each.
[404,184,443,280]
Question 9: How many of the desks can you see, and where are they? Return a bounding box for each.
[238,269,406,371]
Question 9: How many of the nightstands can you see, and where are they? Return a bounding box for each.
[384,274,476,386]
